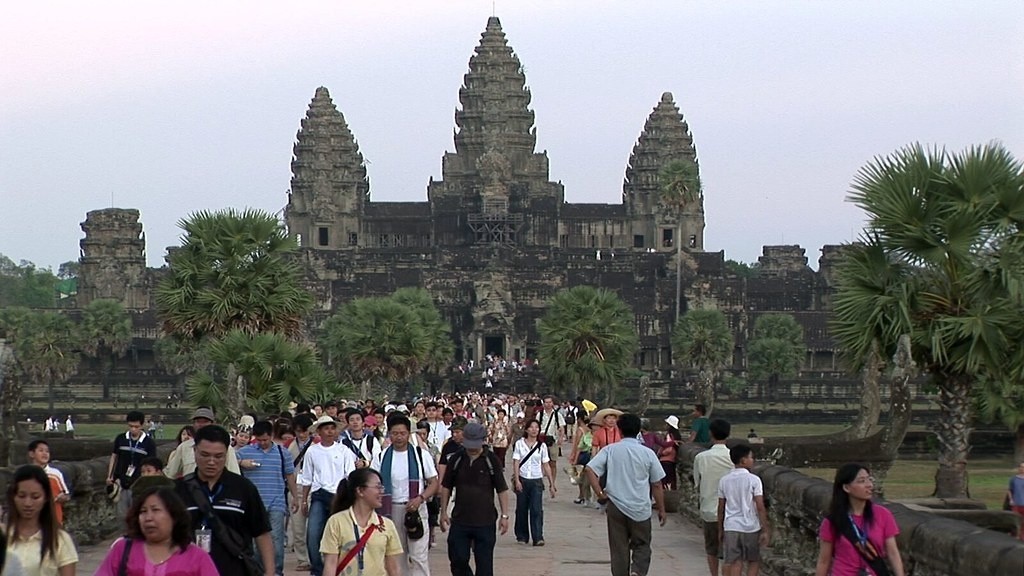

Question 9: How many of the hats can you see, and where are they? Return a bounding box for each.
[665,415,679,429]
[517,411,525,418]
[288,401,298,408]
[307,416,344,436]
[448,416,468,431]
[463,423,484,448]
[346,401,357,409]
[594,408,624,426]
[189,409,214,420]
[404,510,424,539]
[385,404,411,417]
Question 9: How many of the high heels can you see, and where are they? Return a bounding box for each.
[573,499,585,504]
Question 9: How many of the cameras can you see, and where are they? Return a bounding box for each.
[251,462,261,467]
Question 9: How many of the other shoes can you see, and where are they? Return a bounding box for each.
[518,539,526,544]
[593,502,601,509]
[533,540,545,546]
[584,500,593,507]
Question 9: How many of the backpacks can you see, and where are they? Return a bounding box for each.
[578,431,593,450]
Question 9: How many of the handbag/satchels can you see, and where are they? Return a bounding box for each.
[246,553,265,576]
[565,416,575,424]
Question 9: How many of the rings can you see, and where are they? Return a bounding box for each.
[411,506,414,508]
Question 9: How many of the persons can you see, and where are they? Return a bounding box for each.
[457,356,475,375]
[146,419,164,440]
[641,417,684,508]
[27,440,73,530]
[174,424,276,576]
[140,457,165,477]
[370,415,440,576]
[322,468,403,576]
[747,428,758,438]
[440,424,509,575]
[416,421,440,549]
[0,465,76,576]
[302,416,357,576]
[235,421,300,576]
[44,413,61,432]
[717,443,769,575]
[536,395,565,497]
[270,389,598,555]
[479,352,539,389]
[585,414,667,576]
[513,419,556,546]
[694,419,736,576]
[106,410,158,538]
[817,463,906,576]
[92,485,220,576]
[591,409,624,514]
[66,414,75,439]
[289,415,317,572]
[165,408,258,480]
[656,415,681,490]
[685,404,712,443]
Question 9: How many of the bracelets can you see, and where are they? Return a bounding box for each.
[239,459,242,466]
[421,494,429,501]
[597,491,604,496]
[415,494,424,504]
[501,514,509,519]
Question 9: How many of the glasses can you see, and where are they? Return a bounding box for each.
[854,477,876,484]
[362,484,385,492]
